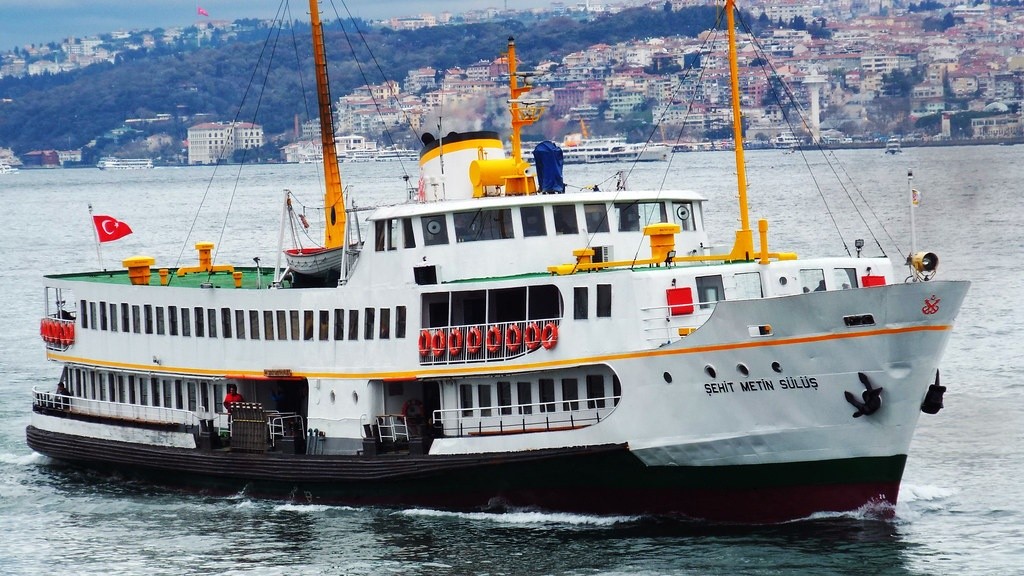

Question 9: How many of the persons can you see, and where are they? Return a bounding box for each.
[223,384,245,414]
[57,381,71,409]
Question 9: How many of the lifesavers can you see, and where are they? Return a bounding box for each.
[486,325,502,352]
[431,330,447,358]
[466,327,483,354]
[400,398,427,422]
[417,329,432,358]
[523,323,542,350]
[39,319,75,345]
[504,324,521,352]
[541,322,559,350]
[448,328,462,356]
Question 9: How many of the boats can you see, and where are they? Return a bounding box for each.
[25,0,972,524]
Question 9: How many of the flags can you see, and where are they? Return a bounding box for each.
[196,6,210,17]
[911,189,922,209]
[90,214,134,244]
